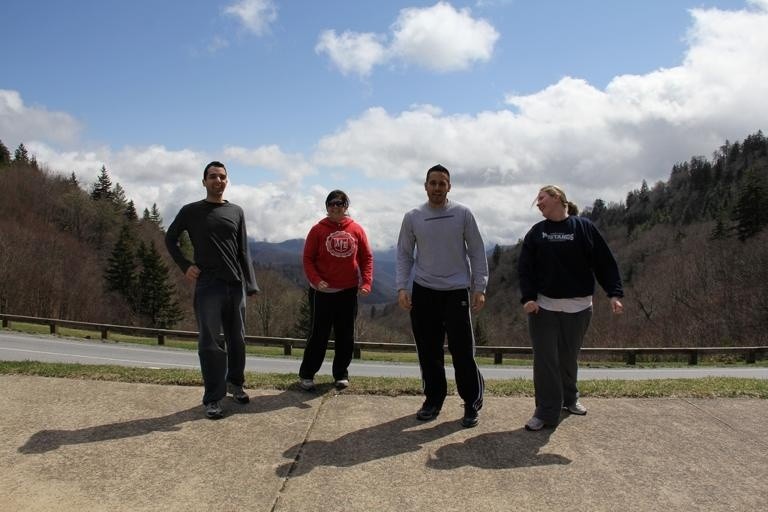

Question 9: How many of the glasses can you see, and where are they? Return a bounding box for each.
[327,201,345,207]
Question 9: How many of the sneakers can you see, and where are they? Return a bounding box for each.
[461,406,478,428]
[226,382,250,405]
[415,404,440,421]
[205,400,224,419]
[567,399,587,416]
[335,379,349,389]
[525,416,544,431]
[300,378,316,390]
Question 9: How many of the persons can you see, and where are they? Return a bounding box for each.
[165,162,259,419]
[397,164,489,428]
[299,190,373,391]
[519,186,624,431]
